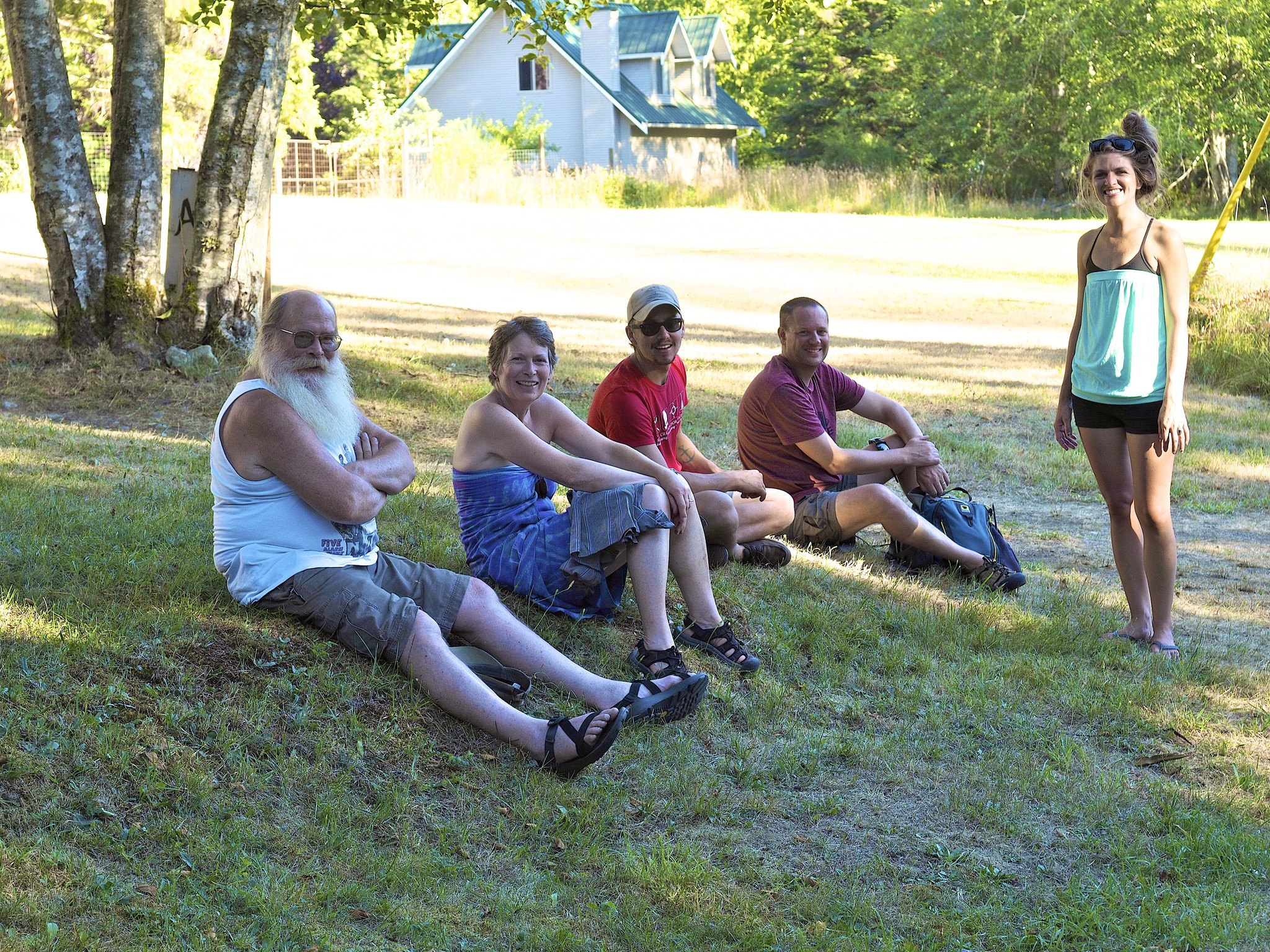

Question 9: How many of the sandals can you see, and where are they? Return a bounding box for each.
[610,672,709,727]
[537,706,629,779]
[674,611,760,672]
[627,638,688,680]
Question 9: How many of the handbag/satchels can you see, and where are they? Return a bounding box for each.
[884,487,1021,575]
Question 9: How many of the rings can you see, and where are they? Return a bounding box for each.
[940,481,946,485]
[688,498,694,503]
[1177,429,1185,434]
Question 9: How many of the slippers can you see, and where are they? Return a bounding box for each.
[1099,630,1136,643]
[1149,641,1180,662]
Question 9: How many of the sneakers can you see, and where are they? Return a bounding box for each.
[706,544,729,571]
[962,555,1027,593]
[740,538,791,568]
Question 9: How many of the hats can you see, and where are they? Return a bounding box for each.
[449,645,532,707]
[627,284,682,326]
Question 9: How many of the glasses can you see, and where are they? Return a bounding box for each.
[868,436,898,483]
[276,328,343,352]
[534,475,547,499]
[1089,138,1138,152]
[630,317,683,336]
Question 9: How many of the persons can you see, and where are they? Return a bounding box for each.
[588,283,797,568]
[1054,108,1192,659]
[451,314,762,690]
[327,443,380,557]
[736,297,1029,591]
[207,290,712,778]
